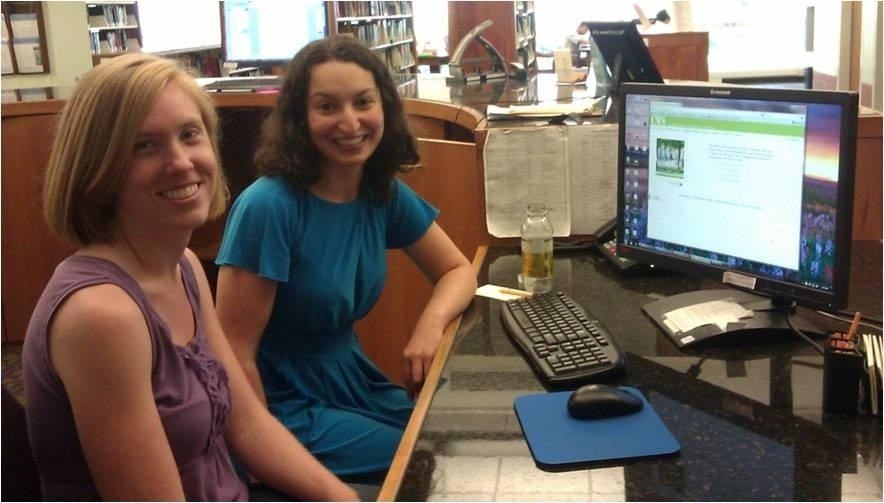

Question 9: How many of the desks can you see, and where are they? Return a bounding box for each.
[376,244,882,503]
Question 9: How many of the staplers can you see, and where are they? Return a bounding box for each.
[509,62,526,80]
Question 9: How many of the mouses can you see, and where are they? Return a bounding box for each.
[567,384,643,420]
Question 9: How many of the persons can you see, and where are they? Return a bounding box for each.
[563,20,590,67]
[213,33,480,488]
[20,51,366,500]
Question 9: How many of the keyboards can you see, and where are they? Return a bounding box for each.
[499,290,624,392]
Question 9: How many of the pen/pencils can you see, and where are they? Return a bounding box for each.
[500,288,530,296]
[845,312,861,339]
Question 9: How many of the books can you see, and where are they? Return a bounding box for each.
[82,2,129,54]
[515,1,541,105]
[0,11,48,103]
[334,0,417,97]
[862,332,882,417]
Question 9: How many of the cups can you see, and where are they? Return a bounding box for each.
[672,0,695,32]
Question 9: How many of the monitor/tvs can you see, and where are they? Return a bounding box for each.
[585,22,665,124]
[617,82,859,353]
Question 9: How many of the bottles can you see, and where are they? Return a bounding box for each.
[520,202,554,293]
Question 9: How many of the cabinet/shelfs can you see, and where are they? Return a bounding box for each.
[87,0,143,65]
[326,0,537,72]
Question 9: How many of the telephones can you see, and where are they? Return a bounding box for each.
[593,216,647,271]
